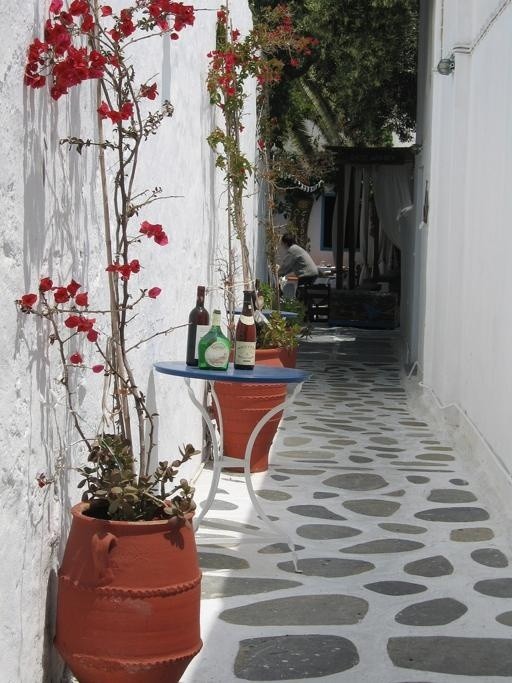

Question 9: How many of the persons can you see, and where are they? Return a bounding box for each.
[276,231,319,323]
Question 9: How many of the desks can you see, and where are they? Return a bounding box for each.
[152,358,312,575]
[213,308,299,329]
[284,275,335,323]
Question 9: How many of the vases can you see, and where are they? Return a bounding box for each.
[54,496,202,683]
[263,344,296,369]
[214,347,288,473]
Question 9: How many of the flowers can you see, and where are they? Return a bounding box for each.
[211,4,307,352]
[250,5,310,348]
[12,0,203,534]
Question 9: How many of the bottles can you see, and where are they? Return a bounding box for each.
[198,309,231,372]
[252,279,264,312]
[235,290,257,370]
[187,285,210,367]
[278,284,284,301]
